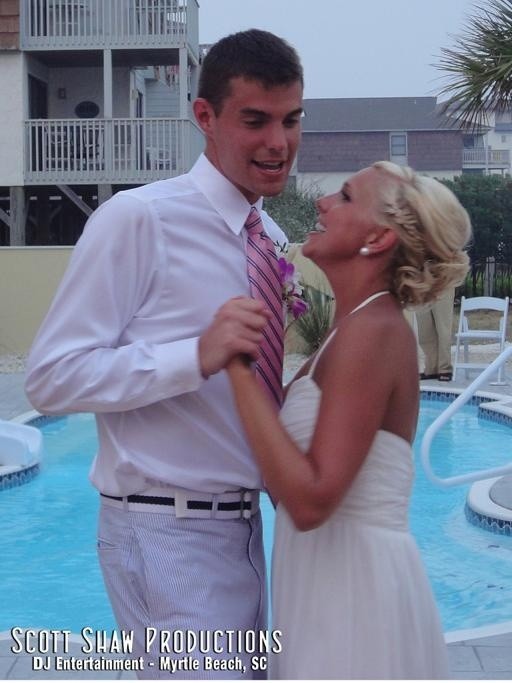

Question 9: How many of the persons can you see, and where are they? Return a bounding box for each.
[21,27,306,679]
[410,283,457,383]
[226,158,474,679]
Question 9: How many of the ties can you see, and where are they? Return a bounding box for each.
[244,207,287,513]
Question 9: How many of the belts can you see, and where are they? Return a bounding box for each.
[99,487,262,523]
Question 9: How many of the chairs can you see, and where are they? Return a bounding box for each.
[446,290,512,388]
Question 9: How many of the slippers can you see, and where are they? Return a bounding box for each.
[438,371,453,382]
[419,372,438,380]
